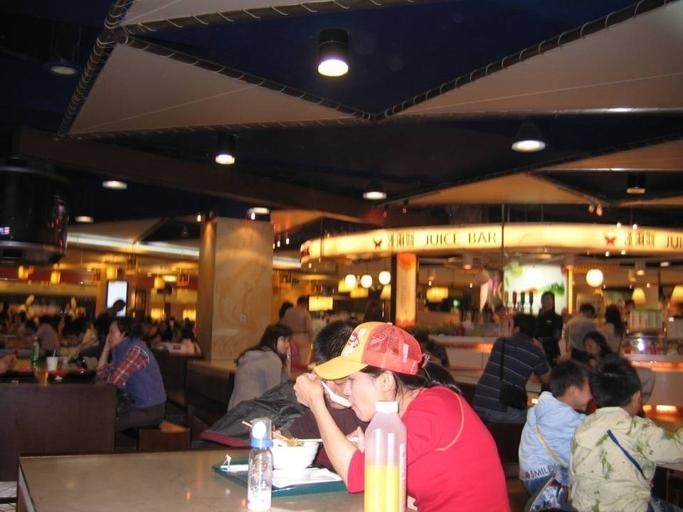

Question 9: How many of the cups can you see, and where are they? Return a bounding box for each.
[32,360,49,384]
[46,356,58,372]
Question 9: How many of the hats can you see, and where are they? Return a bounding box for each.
[313,321,423,380]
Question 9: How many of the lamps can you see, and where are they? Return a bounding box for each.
[214,134,237,165]
[625,173,646,195]
[362,178,387,201]
[317,28,350,77]
[511,122,549,153]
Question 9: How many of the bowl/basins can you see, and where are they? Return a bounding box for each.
[272,438,319,469]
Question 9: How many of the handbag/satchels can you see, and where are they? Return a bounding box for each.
[500,385,528,409]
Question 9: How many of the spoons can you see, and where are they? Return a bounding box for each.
[321,381,352,408]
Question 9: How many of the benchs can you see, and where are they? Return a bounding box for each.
[115,419,191,454]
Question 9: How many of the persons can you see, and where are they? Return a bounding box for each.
[472,291,627,511]
[570,355,683,512]
[293,321,513,511]
[0,354,16,378]
[227,295,451,471]
[1,302,200,357]
[98,316,167,430]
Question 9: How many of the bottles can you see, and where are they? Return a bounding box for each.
[247,418,272,512]
[79,360,89,378]
[31,336,41,368]
[364,399,407,512]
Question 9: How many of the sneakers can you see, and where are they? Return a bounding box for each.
[524,476,561,512]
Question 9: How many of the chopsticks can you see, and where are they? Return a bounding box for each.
[242,419,289,442]
